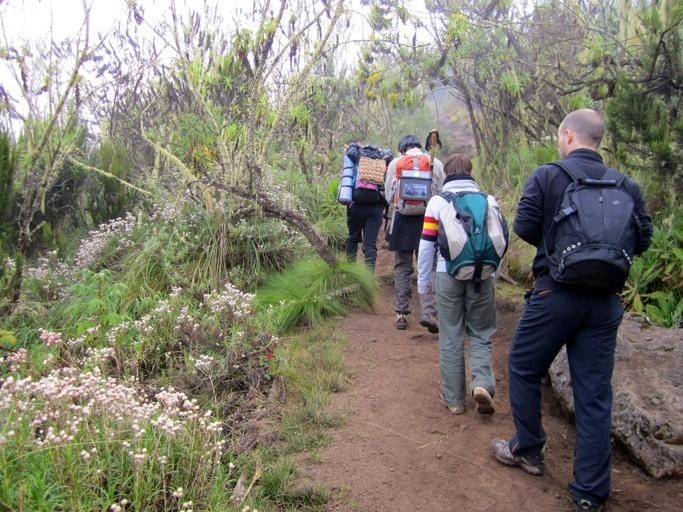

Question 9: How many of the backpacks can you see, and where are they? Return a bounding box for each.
[539,158,643,297]
[391,152,432,216]
[336,143,393,232]
[432,189,509,293]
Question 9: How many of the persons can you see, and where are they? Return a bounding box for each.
[384,135,445,333]
[418,156,510,415]
[488,108,654,511]
[340,141,394,274]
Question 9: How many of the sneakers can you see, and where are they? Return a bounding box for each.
[473,387,495,414]
[419,315,439,333]
[572,498,604,512]
[489,439,545,475]
[395,317,408,330]
[441,392,464,414]
